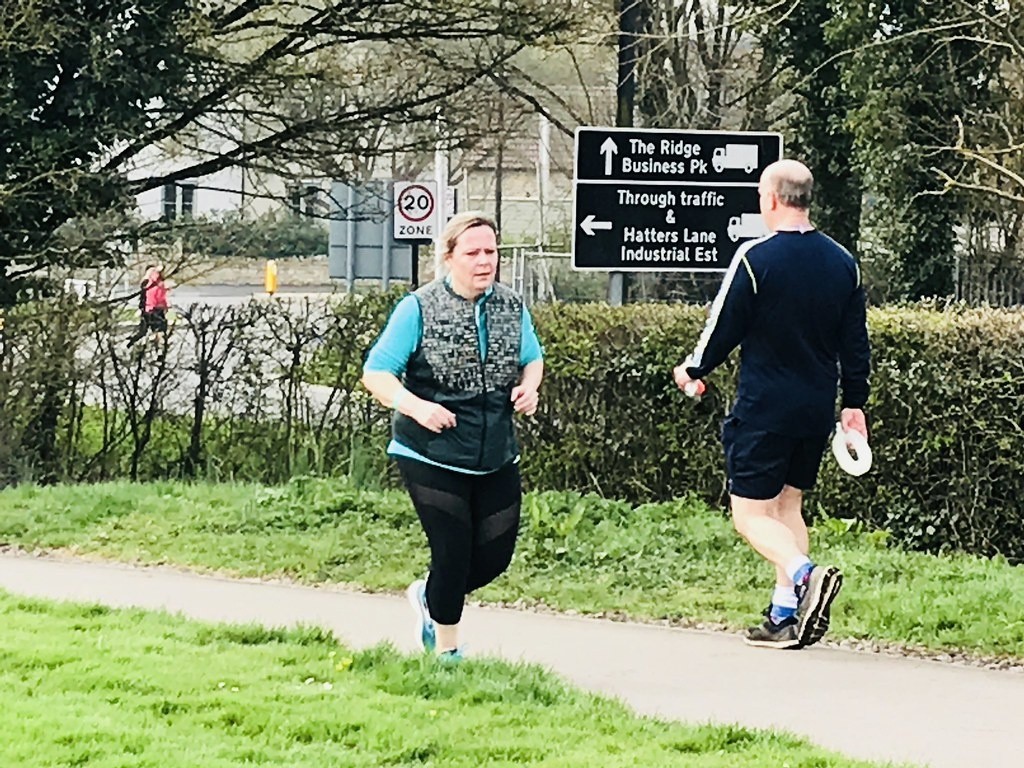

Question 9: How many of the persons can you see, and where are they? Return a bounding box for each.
[123,260,174,365]
[363,211,545,670]
[672,159,873,646]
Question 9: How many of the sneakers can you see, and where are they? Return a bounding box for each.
[794,563,843,647]
[743,602,799,648]
[436,647,462,667]
[407,580,435,654]
